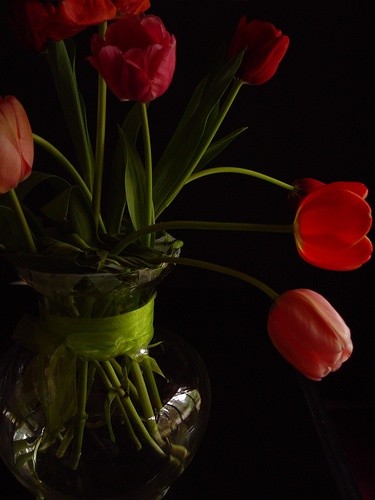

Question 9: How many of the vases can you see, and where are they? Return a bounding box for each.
[1,266,212,499]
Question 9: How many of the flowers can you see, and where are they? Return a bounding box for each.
[0,1,373,471]
